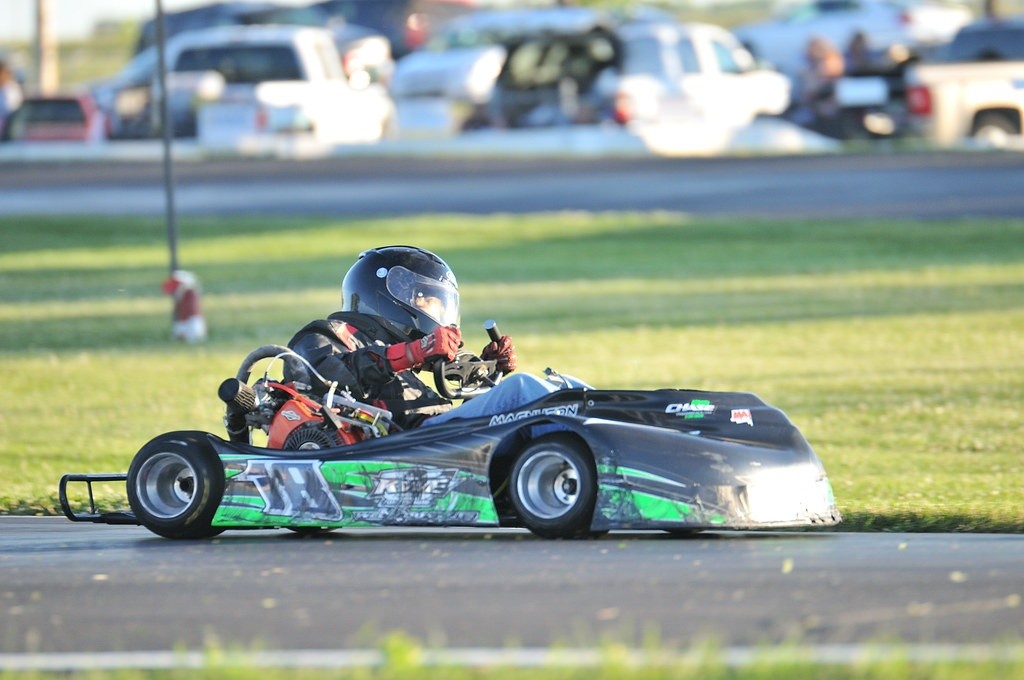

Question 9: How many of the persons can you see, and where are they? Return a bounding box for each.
[280,244,517,428]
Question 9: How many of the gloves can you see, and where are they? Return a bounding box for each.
[409,326,461,368]
[479,335,518,377]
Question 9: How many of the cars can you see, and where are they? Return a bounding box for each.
[10,93,105,142]
[109,0,1024,154]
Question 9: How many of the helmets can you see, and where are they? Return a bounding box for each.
[340,244,461,336]
